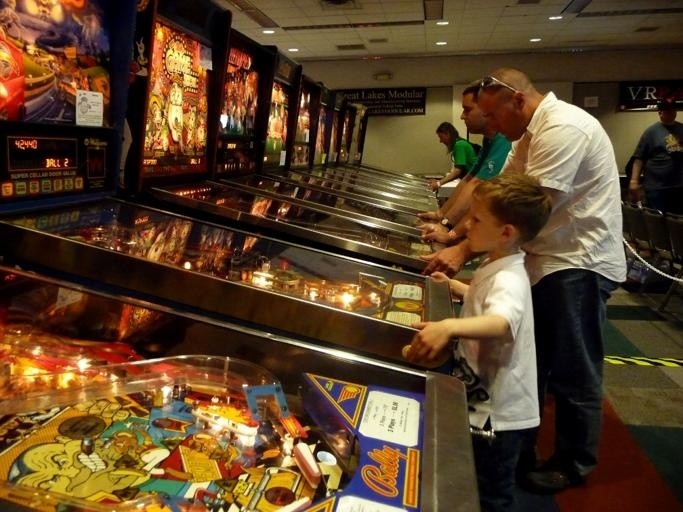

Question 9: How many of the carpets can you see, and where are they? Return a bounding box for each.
[536,387,682,511]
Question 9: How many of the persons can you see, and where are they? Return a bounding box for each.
[426,121,477,191]
[419,66,629,495]
[404,172,555,512]
[623,98,681,261]
[414,81,514,237]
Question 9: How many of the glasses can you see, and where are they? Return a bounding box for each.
[477,73,518,94]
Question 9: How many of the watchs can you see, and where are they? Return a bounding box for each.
[439,217,453,230]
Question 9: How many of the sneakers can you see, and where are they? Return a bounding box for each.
[523,458,587,490]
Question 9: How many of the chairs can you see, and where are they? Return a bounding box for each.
[621,201,682,312]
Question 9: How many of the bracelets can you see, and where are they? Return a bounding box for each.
[436,180,441,187]
[629,178,639,185]
[446,229,459,241]
